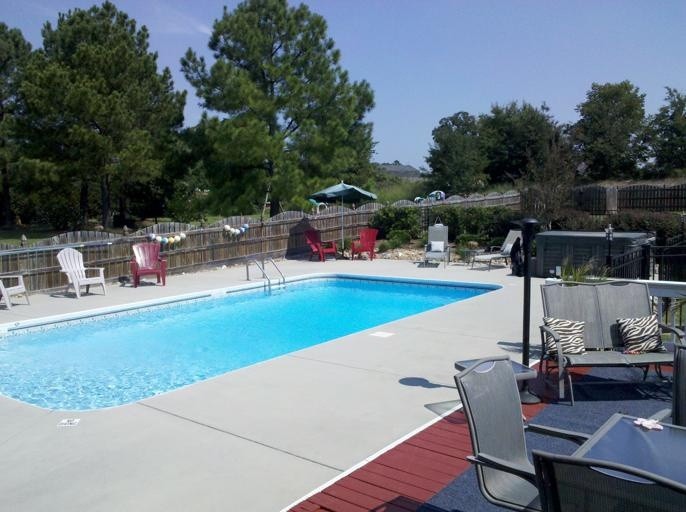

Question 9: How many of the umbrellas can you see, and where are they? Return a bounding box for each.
[306,180,377,252]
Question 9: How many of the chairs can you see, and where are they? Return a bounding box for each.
[529,448,686,511]
[467,228,524,270]
[128,242,169,288]
[302,227,337,264]
[351,228,379,260]
[54,247,108,297]
[452,353,592,511]
[423,225,451,269]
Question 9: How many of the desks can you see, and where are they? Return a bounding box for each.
[570,412,686,495]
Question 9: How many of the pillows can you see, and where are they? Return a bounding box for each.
[501,242,514,255]
[430,240,445,252]
[616,313,666,353]
[543,316,587,353]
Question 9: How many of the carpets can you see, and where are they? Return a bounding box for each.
[413,353,686,510]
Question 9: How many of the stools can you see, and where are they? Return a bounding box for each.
[0,274,31,312]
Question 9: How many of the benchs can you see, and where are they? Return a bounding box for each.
[539,276,685,405]
